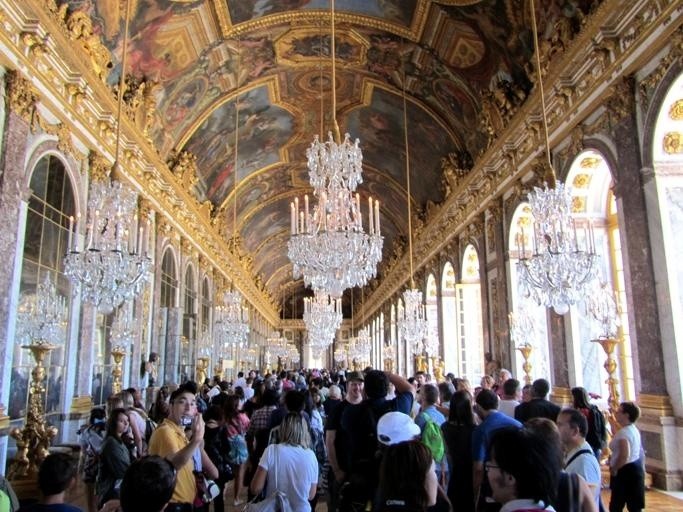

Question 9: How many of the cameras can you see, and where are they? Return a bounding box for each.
[181,416,192,426]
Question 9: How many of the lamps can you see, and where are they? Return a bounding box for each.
[352,344,427,375]
[215,216,250,352]
[67,92,154,317]
[510,48,598,318]
[505,306,539,393]
[597,275,624,451]
[215,354,271,379]
[332,298,374,360]
[268,297,302,363]
[287,18,385,298]
[397,138,432,344]
[195,333,208,384]
[293,287,344,361]
[104,304,143,401]
[7,270,71,497]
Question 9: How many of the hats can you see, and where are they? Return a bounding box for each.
[347,372,364,382]
[376,411,421,446]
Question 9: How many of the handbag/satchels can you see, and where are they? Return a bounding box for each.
[199,473,220,503]
[243,490,292,512]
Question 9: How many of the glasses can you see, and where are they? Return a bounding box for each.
[485,462,500,471]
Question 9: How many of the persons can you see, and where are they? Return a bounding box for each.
[29,419,60,463]
[77,354,645,511]
[27,451,84,510]
[9,427,30,477]
